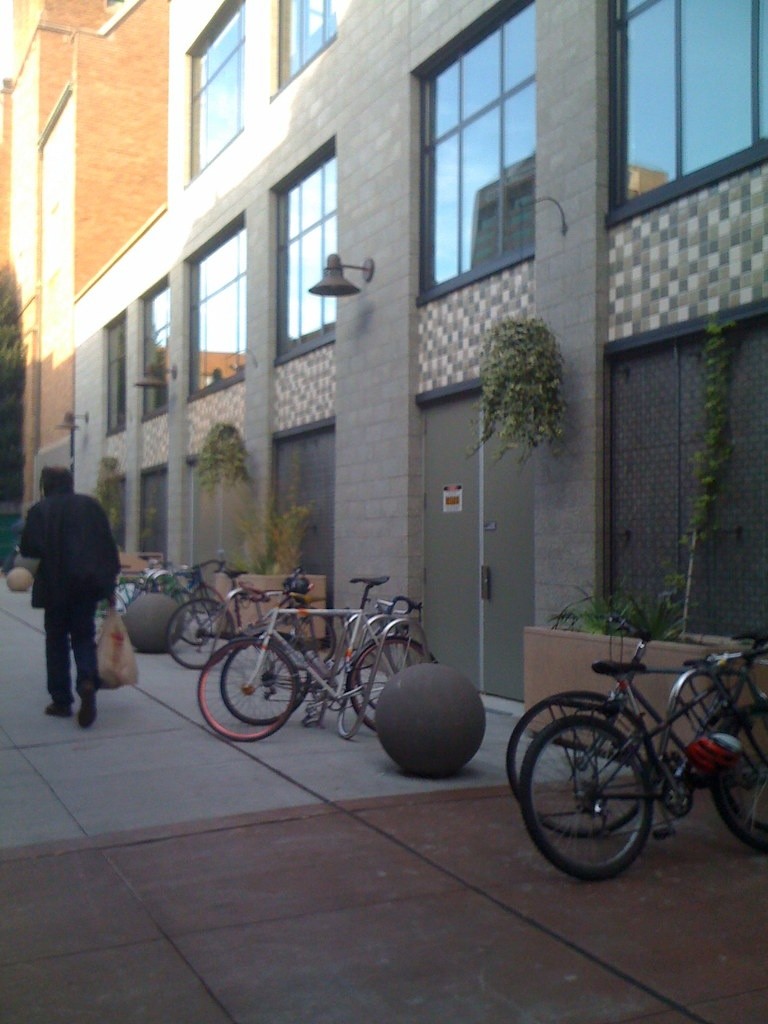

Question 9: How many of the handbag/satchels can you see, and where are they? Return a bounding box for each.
[96,604,139,688]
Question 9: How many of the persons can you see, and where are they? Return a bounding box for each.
[20,466,121,728]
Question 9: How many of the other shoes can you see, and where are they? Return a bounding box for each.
[44,703,73,717]
[78,683,98,728]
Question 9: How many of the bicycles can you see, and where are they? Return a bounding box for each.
[507,617,768,884]
[93,553,439,744]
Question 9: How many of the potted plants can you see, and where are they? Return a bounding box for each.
[524,313,768,772]
[217,448,328,642]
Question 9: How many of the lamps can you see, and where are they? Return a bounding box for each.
[54,411,88,430]
[309,254,375,296]
[133,364,178,387]
